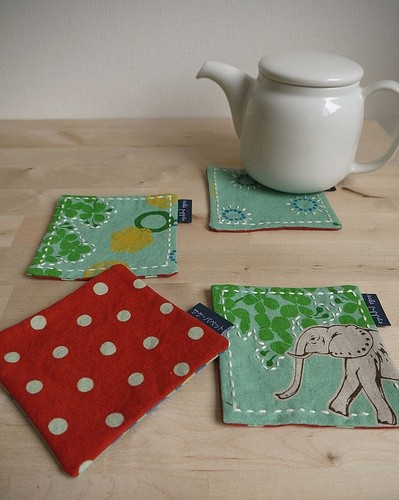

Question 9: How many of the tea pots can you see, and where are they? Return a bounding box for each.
[196,50,399,194]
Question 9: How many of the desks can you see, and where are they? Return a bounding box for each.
[1,115,398,499]
